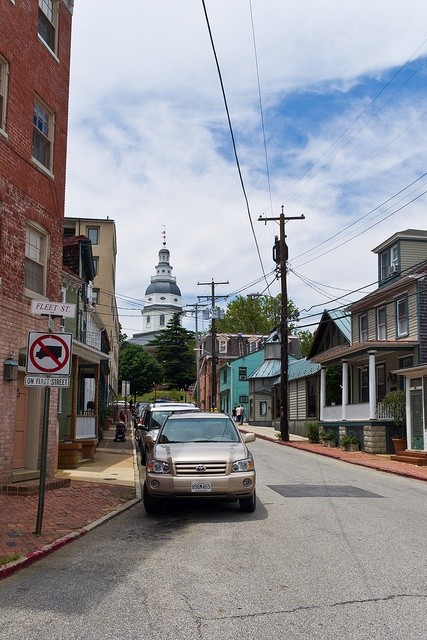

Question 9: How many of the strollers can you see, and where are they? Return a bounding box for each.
[114,421,127,442]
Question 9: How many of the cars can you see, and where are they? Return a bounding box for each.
[111,401,132,409]
[133,402,148,416]
[139,406,202,464]
[143,413,257,513]
[146,403,196,410]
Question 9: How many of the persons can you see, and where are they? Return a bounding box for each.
[129,399,133,410]
[232,404,245,426]
[117,411,127,433]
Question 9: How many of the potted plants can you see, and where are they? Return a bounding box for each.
[304,422,318,443]
[382,390,406,455]
[320,430,326,446]
[339,436,349,450]
[349,433,359,452]
[326,430,337,446]
[102,404,111,430]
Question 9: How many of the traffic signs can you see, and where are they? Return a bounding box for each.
[26,297,82,321]
